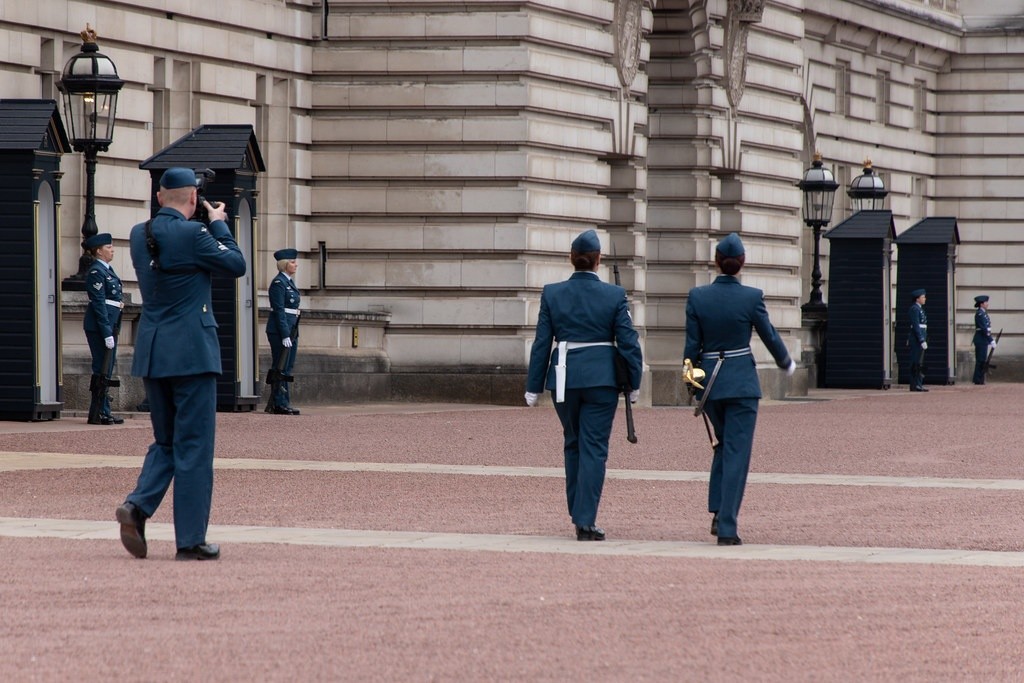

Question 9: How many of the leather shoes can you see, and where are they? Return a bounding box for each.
[576,526,605,540]
[87,414,124,425]
[116,501,147,559]
[176,544,220,561]
[710,516,720,536]
[910,386,929,392]
[264,407,300,415]
[717,535,742,545]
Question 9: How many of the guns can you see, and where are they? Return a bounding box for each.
[87,309,125,424]
[611,239,639,444]
[264,314,302,414]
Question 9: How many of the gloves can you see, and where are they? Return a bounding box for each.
[682,364,694,373]
[105,336,115,349]
[630,389,639,402]
[524,391,538,407]
[282,337,292,347]
[991,341,996,348]
[921,342,927,350]
[787,360,796,376]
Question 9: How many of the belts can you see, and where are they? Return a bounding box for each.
[911,324,927,329]
[556,341,616,350]
[976,327,991,331]
[270,307,301,315]
[702,347,751,359]
[89,299,124,308]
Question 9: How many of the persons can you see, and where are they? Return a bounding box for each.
[265,248,301,415]
[683,232,796,546]
[971,295,996,385]
[115,167,247,561]
[905,288,929,391]
[83,233,125,424]
[524,230,643,541]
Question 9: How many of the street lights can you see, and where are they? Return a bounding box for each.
[795,148,841,313]
[54,24,125,291]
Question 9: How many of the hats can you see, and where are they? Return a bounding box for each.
[160,167,198,189]
[572,230,600,252]
[86,233,112,249]
[974,295,989,302]
[274,248,297,261]
[911,289,927,297]
[717,233,745,257]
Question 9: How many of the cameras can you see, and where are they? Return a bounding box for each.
[192,168,219,223]
